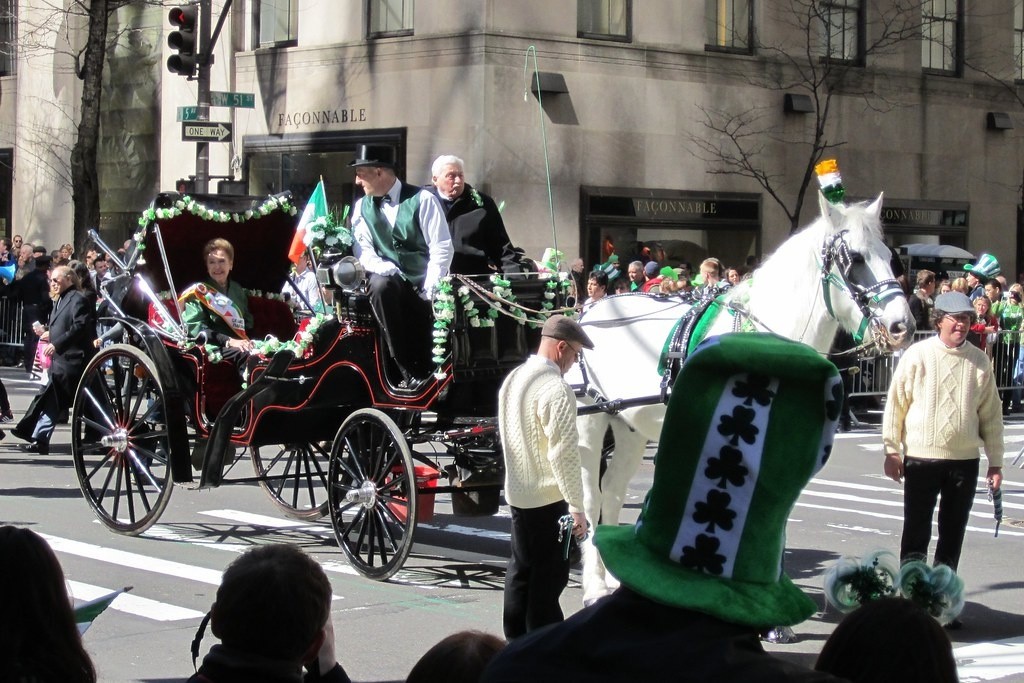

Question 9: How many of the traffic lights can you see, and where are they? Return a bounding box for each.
[166,5,198,76]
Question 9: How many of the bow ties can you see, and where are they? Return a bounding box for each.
[372,193,392,208]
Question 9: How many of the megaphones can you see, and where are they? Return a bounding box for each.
[0,263,15,283]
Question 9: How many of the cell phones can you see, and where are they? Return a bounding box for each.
[32,321,44,333]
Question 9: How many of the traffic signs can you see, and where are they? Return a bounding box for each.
[211,92,256,110]
[182,123,233,143]
[177,104,200,122]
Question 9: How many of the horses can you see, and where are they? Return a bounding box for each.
[568,192,920,609]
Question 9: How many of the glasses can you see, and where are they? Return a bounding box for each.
[49,276,64,283]
[941,313,974,325]
[558,339,581,361]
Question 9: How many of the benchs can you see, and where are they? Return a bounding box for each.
[146,292,294,367]
[247,328,377,377]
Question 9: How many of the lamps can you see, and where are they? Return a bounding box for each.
[986,112,1015,130]
[530,71,570,93]
[783,93,816,113]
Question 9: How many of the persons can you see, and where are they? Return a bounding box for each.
[828,254,1024,430]
[281,253,319,310]
[185,545,351,683]
[405,333,966,683]
[349,143,527,387]
[883,293,1002,625]
[0,235,131,455]
[0,525,97,683]
[499,314,594,641]
[536,247,740,300]
[181,237,283,413]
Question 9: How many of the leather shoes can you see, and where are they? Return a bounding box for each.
[17,439,49,454]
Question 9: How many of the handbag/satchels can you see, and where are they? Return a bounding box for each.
[39,335,52,368]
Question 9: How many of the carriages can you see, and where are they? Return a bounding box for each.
[69,159,918,582]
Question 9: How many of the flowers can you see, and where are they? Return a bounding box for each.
[180,342,223,365]
[308,203,353,261]
[159,285,295,311]
[132,191,305,265]
[249,311,326,360]
[432,270,575,379]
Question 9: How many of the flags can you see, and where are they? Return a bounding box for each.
[75,589,121,636]
[289,181,326,264]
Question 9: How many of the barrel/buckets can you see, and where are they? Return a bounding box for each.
[444,464,500,517]
[386,466,439,524]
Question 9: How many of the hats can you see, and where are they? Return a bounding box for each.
[345,143,397,170]
[935,293,974,315]
[963,254,1002,281]
[34,256,53,267]
[646,260,659,275]
[541,314,594,351]
[592,331,847,626]
[33,246,46,254]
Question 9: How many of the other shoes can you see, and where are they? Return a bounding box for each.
[0,410,14,424]
[407,376,427,390]
[11,428,37,442]
[1001,403,1011,416]
[944,619,962,629]
[132,390,138,396]
[56,410,70,424]
